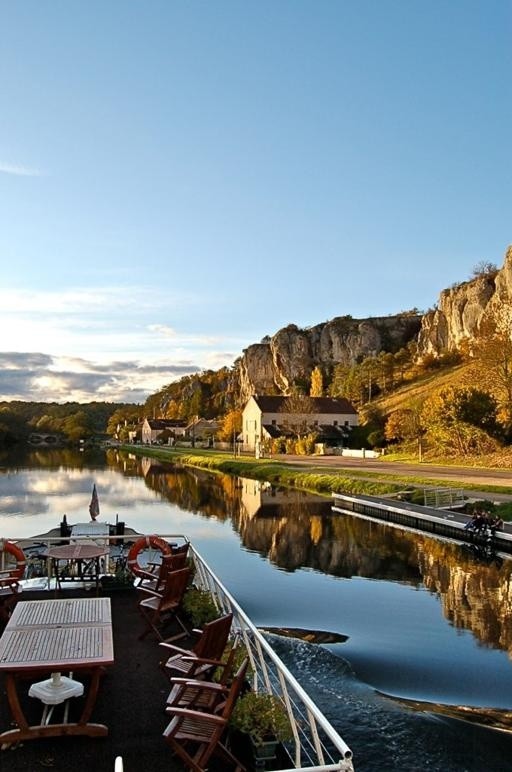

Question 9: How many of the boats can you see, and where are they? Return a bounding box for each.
[0,481,365,772]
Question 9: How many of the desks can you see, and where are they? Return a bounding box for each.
[0,543,116,743]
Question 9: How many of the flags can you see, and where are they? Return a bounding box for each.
[88,485,100,520]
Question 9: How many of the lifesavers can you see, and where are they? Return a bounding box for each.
[127,536,171,579]
[0,541,25,587]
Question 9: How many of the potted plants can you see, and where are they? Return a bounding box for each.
[232,690,294,761]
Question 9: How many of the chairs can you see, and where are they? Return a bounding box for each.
[132,540,250,772]
